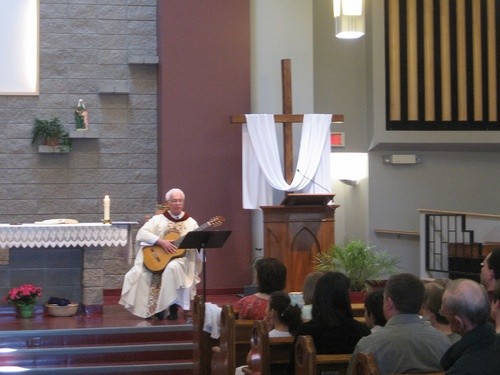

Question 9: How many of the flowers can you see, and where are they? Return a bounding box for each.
[2,284,42,305]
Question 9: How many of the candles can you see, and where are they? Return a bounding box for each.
[103,195,111,220]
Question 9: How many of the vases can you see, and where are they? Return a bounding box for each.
[17,302,34,319]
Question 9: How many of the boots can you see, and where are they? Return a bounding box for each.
[167,304,178,320]
[154,313,163,320]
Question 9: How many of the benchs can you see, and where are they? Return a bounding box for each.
[193,295,380,375]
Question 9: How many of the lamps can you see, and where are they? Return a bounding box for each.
[333,0,365,39]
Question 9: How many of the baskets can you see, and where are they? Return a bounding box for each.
[45,302,78,317]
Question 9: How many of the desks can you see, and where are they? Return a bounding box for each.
[0,224,128,315]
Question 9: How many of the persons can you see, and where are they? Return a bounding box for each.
[233,247,500,375]
[118,188,207,320]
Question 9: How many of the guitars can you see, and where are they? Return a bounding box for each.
[140,215,226,273]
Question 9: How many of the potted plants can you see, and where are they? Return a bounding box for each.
[32,117,73,153]
[312,239,407,304]
